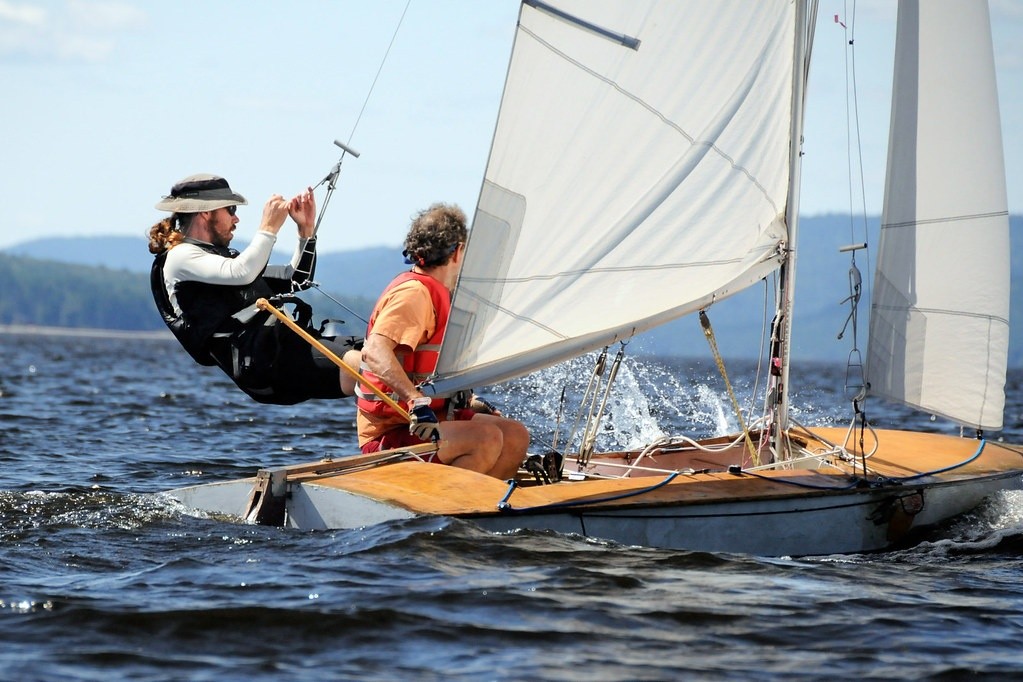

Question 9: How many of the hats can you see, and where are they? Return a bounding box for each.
[155,173,248,213]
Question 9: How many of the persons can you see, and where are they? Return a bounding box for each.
[147,174,364,405]
[355,204,530,482]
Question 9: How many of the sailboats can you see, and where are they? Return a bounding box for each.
[158,0,1023,558]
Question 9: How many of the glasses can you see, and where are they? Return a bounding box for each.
[214,205,236,216]
